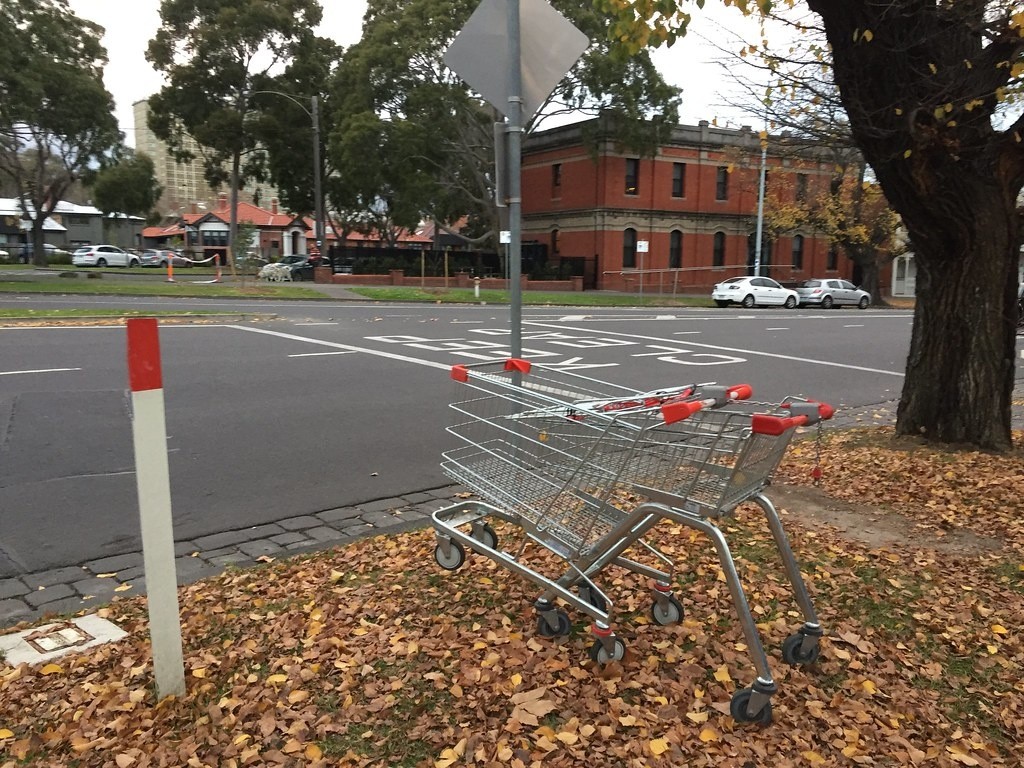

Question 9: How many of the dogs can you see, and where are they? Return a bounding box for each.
[273,265,293,283]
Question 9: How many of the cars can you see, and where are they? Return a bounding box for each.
[796,279,872,309]
[711,276,800,310]
[237,251,364,282]
[0,243,193,268]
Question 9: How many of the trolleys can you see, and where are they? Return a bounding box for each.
[430,357,834,730]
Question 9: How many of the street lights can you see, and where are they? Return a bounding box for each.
[247,90,326,268]
